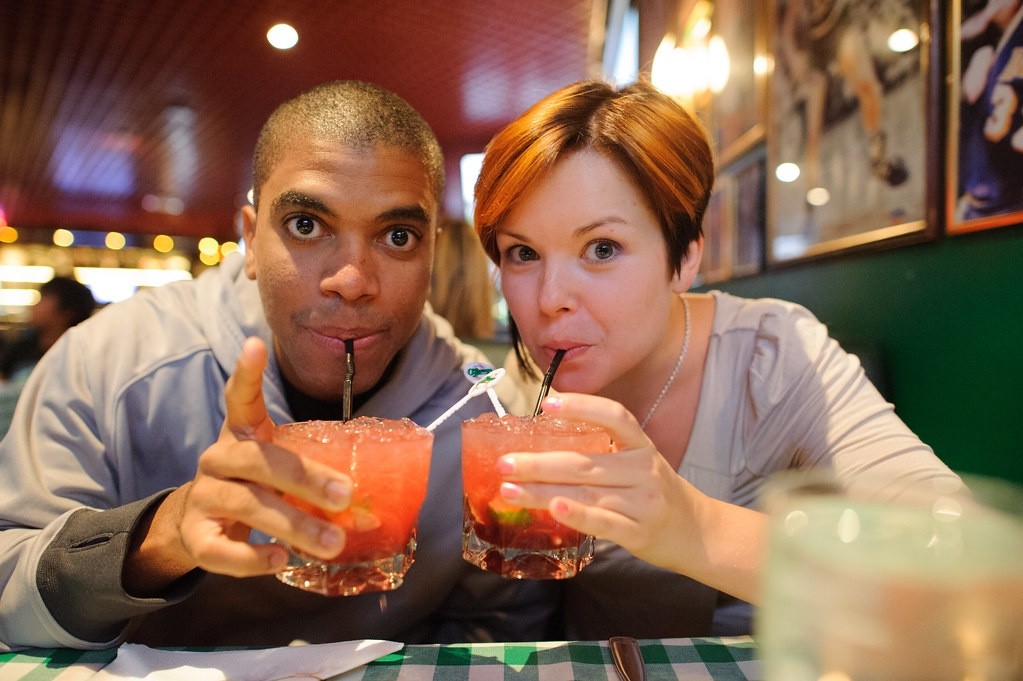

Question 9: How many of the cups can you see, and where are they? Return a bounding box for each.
[271,419,434,597]
[461,418,612,580]
[755,466,1023,681]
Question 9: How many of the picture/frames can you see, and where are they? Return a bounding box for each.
[632,0,1023,294]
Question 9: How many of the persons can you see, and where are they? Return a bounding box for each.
[1,277,96,380]
[957,0,1023,221]
[472,78,973,639]
[0,81,554,649]
[771,0,910,230]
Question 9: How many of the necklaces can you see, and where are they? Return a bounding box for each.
[641,295,692,430]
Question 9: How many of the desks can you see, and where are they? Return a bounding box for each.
[0,631,764,681]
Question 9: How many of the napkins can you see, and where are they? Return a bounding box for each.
[97,640,404,681]
